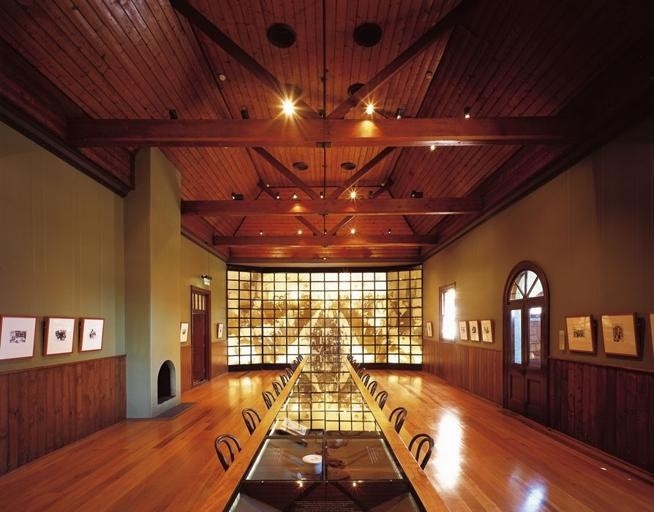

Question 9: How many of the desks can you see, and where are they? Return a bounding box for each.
[198,354,449,511]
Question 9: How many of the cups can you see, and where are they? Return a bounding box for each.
[304,455,323,474]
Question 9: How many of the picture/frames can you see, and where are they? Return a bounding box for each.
[0,315,105,361]
[180,322,189,343]
[564,311,653,361]
[217,323,224,339]
[426,321,433,338]
[457,319,494,345]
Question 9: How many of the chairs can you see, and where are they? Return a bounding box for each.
[215,354,435,474]
